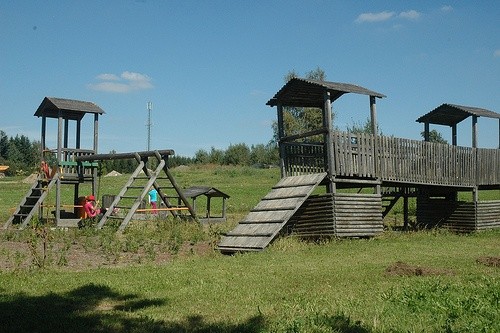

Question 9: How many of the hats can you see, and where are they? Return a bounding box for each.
[87,195,95,201]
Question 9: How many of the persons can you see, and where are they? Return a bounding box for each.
[83,195,109,218]
[147,185,158,216]
